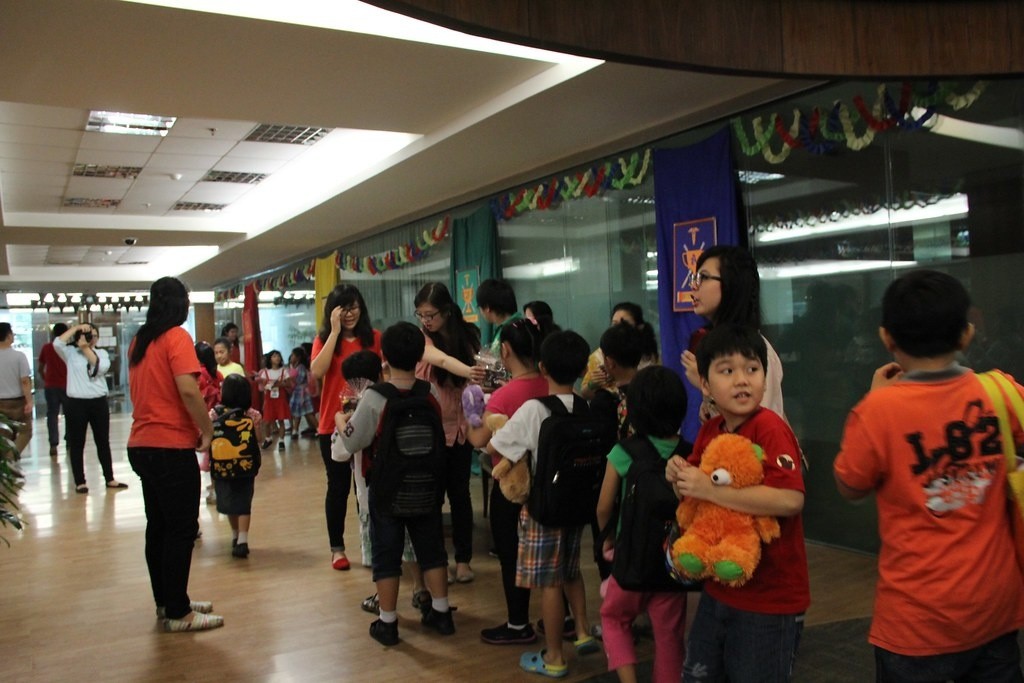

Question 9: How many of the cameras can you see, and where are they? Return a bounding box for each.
[83,332,92,343]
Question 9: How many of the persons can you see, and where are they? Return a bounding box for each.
[127,277,224,632]
[193,244,809,683]
[52,322,128,493]
[38,323,72,455]
[0,322,33,478]
[834,269,1024,683]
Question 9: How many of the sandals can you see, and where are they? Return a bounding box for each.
[519,649,568,677]
[574,636,600,656]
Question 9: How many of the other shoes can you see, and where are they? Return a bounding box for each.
[361,590,431,615]
[332,555,350,569]
[447,562,474,584]
[480,622,536,644]
[261,440,285,450]
[273,426,319,440]
[590,623,654,644]
[75,481,128,493]
[206,484,216,504]
[50,445,57,455]
[232,538,249,558]
[536,617,576,638]
[157,601,223,632]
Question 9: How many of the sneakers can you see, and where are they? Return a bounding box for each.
[369,618,398,646]
[421,606,455,635]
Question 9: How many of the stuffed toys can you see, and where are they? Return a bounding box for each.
[486,413,530,503]
[671,433,779,587]
[462,385,485,427]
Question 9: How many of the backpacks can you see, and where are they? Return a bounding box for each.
[528,392,606,526]
[593,432,694,591]
[201,371,222,412]
[305,370,318,395]
[367,378,446,519]
[210,405,260,481]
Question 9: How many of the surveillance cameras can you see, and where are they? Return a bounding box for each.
[124,238,137,245]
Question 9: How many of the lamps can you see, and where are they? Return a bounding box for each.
[501,200,581,280]
[747,191,970,281]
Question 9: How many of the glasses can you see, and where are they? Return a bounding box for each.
[340,305,359,316]
[413,310,440,321]
[690,272,720,287]
[11,333,16,336]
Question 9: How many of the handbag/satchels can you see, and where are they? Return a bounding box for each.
[974,372,1024,571]
